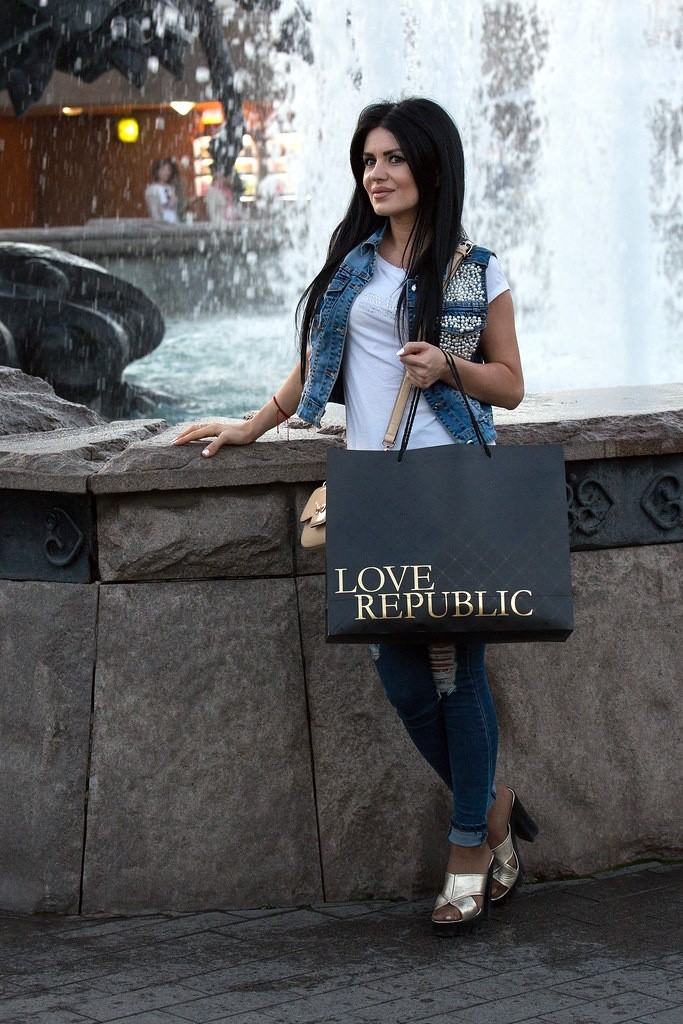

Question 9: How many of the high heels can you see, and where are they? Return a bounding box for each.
[430,847,496,941]
[485,786,542,908]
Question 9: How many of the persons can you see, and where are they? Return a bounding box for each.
[170,95,538,938]
[143,157,248,222]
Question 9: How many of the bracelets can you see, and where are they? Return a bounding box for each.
[272,396,290,441]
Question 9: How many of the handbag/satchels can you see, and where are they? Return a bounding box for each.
[296,349,574,643]
